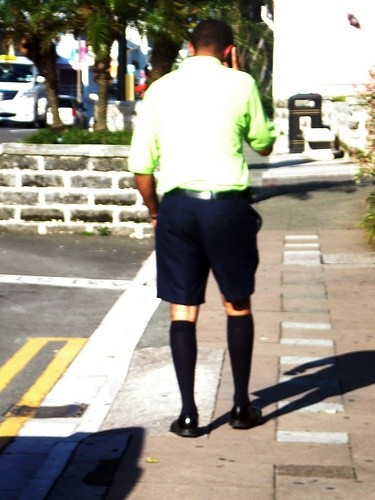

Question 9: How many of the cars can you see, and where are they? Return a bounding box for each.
[45,94,88,129]
[0,54,49,130]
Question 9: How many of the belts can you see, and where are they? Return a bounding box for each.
[184,188,240,200]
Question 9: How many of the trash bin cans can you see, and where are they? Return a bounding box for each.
[288,93,322,153]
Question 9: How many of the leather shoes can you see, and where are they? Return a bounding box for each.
[170,412,198,437]
[230,406,262,429]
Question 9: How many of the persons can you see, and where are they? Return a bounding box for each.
[131,60,151,100]
[127,18,277,438]
